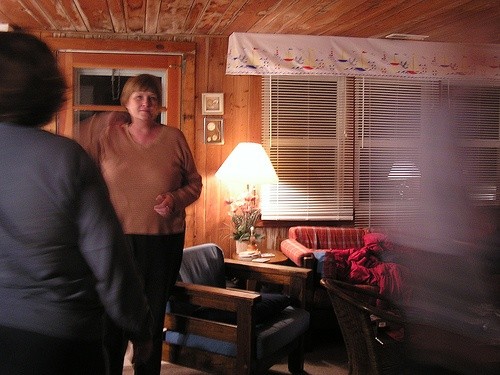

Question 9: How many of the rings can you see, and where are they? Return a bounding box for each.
[166,206,170,210]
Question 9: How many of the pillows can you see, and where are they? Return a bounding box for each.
[191,293,295,326]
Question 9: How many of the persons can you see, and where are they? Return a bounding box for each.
[0,31,156,375]
[82,73,203,375]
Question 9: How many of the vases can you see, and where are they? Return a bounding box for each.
[236,241,249,254]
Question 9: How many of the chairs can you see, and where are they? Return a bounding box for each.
[321,278,437,375]
[163,243,312,374]
[280,226,391,352]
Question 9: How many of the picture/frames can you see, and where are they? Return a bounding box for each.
[204,117,224,145]
[202,92,224,115]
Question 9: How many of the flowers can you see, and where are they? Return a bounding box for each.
[222,194,265,245]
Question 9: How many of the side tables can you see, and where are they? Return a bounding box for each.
[232,249,289,265]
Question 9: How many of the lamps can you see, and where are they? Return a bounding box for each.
[215,143,278,194]
[388,161,421,200]
